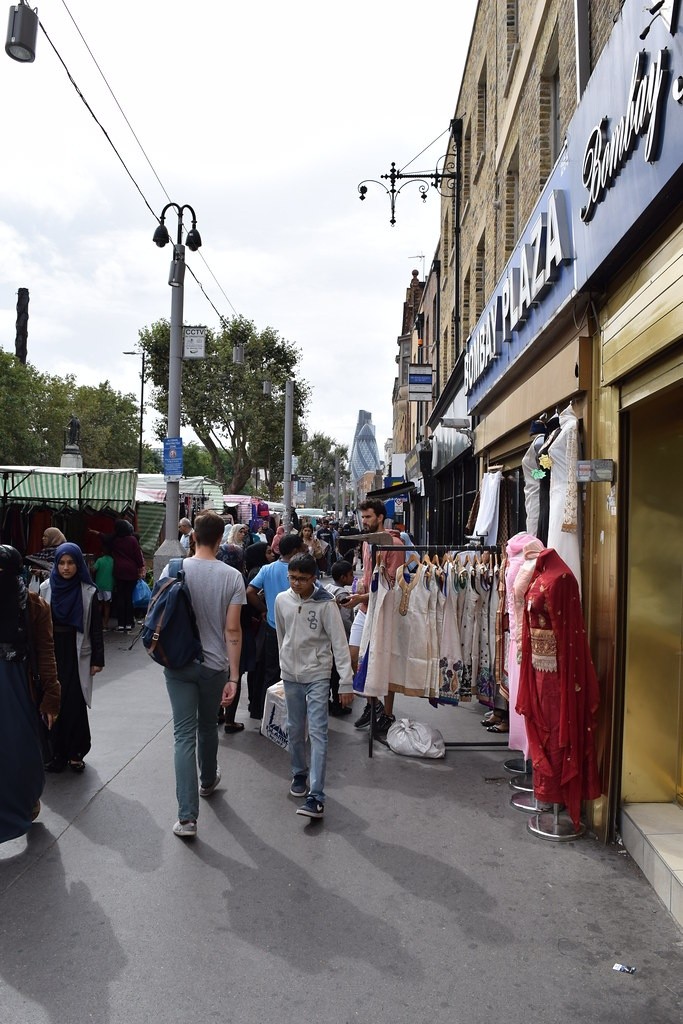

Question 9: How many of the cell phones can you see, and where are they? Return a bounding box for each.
[339,598,350,604]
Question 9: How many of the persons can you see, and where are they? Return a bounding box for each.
[159,511,248,836]
[275,551,354,818]
[217,520,356,576]
[504,531,603,832]
[339,498,406,736]
[40,542,105,773]
[324,560,355,715]
[88,519,147,634]
[218,541,275,734]
[0,545,62,844]
[179,518,195,552]
[522,404,581,598]
[246,534,305,684]
[26,527,67,570]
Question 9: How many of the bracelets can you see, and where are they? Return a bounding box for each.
[228,680,238,684]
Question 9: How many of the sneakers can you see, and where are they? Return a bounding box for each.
[172,820,197,836]
[296,795,325,818]
[198,764,221,796]
[373,713,397,736]
[290,772,307,796]
[354,700,385,728]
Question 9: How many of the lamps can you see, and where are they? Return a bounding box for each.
[168,253,185,287]
[302,433,307,442]
[264,380,272,394]
[233,346,244,365]
[5,4,38,62]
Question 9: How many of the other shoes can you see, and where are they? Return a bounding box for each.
[66,759,86,771]
[224,723,244,734]
[42,751,57,767]
[124,625,133,633]
[32,799,40,821]
[216,714,226,725]
[102,626,111,632]
[112,625,124,633]
[487,721,510,733]
[328,700,352,717]
[481,715,504,726]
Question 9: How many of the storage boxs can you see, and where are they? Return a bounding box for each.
[261,680,308,752]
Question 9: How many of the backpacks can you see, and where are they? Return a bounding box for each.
[140,560,205,669]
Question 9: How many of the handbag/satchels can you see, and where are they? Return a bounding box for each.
[132,580,152,607]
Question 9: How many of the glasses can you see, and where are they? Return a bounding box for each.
[286,574,315,584]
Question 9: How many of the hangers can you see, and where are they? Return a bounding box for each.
[377,546,388,573]
[6,498,135,520]
[488,463,505,473]
[403,545,498,582]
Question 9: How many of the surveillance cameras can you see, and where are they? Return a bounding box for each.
[186,229,202,252]
[437,416,471,429]
[152,225,169,248]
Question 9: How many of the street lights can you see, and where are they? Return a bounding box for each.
[123,352,144,471]
[152,202,202,585]
